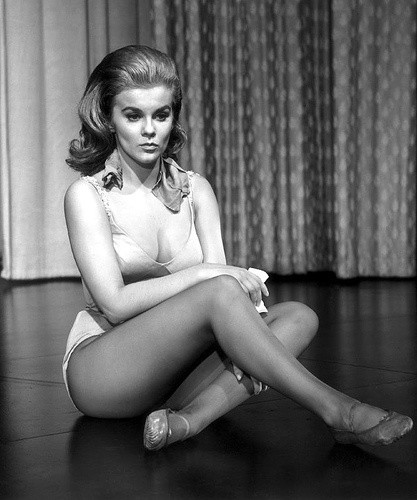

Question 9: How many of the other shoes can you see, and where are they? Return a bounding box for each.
[333,401,413,446]
[143,408,190,451]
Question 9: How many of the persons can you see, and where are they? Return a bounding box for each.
[62,43,413,450]
